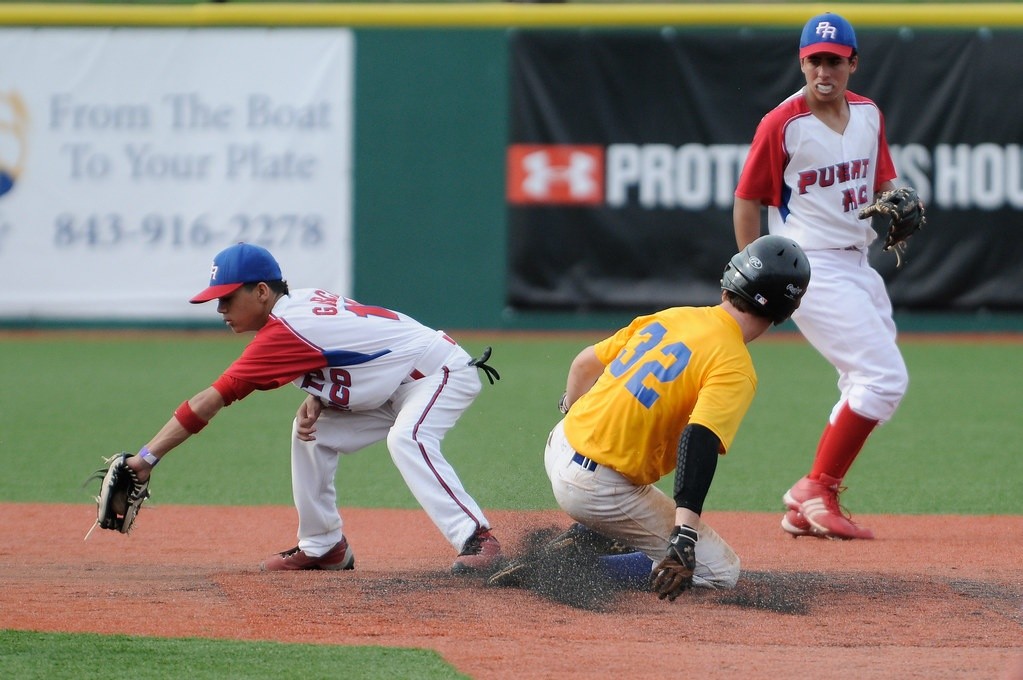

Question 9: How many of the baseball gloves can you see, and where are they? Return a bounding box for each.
[858,186,927,253]
[96,451,152,535]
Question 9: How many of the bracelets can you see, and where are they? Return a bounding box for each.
[142,448,159,467]
[174,400,208,435]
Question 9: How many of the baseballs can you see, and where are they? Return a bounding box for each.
[110,489,128,515]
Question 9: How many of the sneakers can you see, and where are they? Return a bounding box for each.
[487,538,581,588]
[781,509,875,540]
[782,473,855,539]
[451,525,502,577]
[546,523,609,556]
[260,535,355,572]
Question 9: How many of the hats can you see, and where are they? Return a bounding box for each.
[799,11,857,58]
[189,241,282,304]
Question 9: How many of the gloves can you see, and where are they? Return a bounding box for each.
[648,523,699,602]
[467,345,500,385]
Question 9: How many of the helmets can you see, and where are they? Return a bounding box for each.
[720,234,811,328]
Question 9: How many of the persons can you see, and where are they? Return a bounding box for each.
[732,13,925,539]
[486,233,812,603]
[96,241,505,577]
